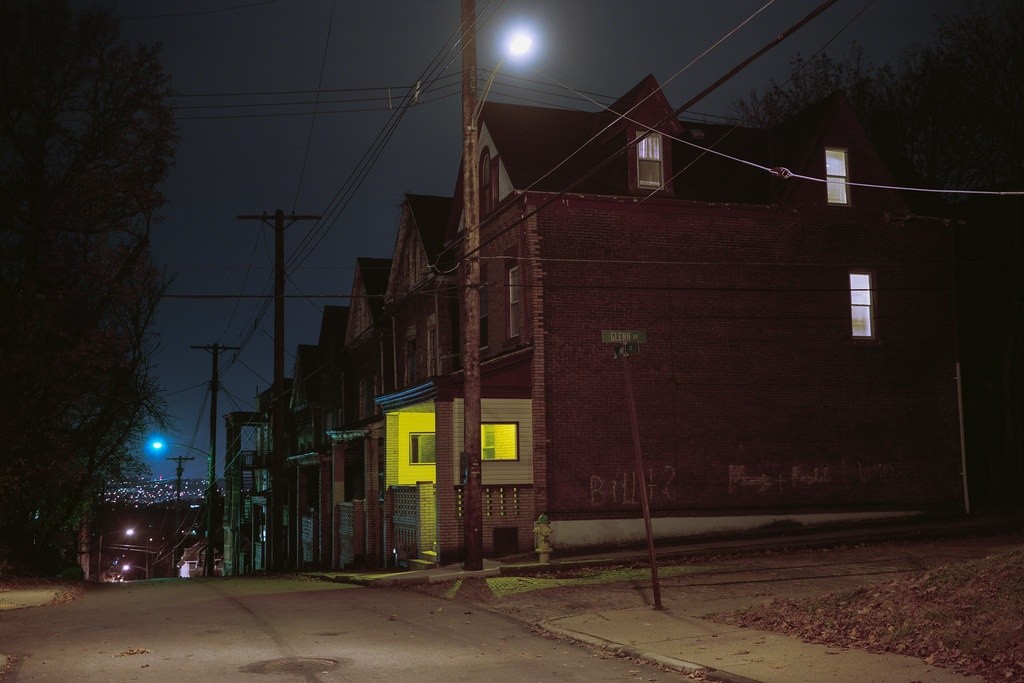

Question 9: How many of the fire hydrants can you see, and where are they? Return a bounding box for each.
[531,513,554,565]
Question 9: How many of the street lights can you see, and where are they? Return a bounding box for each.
[122,564,149,580]
[151,441,216,577]
[95,527,136,584]
[462,28,535,570]
